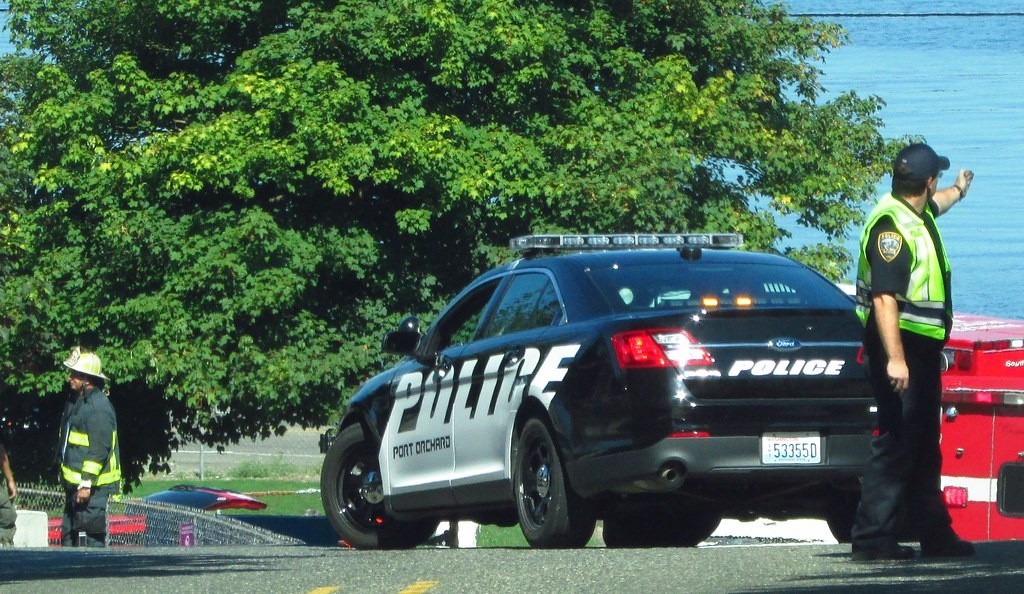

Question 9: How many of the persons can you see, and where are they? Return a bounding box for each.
[57,347,121,546]
[851,144,976,561]
[0,439,18,548]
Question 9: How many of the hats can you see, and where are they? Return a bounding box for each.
[63,347,111,382]
[893,142,950,178]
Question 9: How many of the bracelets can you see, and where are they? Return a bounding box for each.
[953,184,964,201]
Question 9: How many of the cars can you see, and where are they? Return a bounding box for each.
[319,231,880,549]
[48,485,267,550]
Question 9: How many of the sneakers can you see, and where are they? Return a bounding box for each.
[920,528,976,558]
[852,543,914,560]
